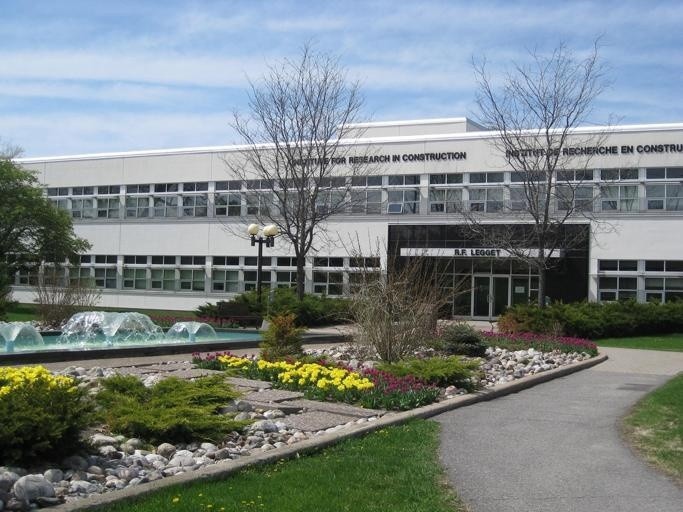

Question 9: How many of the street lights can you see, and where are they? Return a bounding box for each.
[248,223,277,302]
